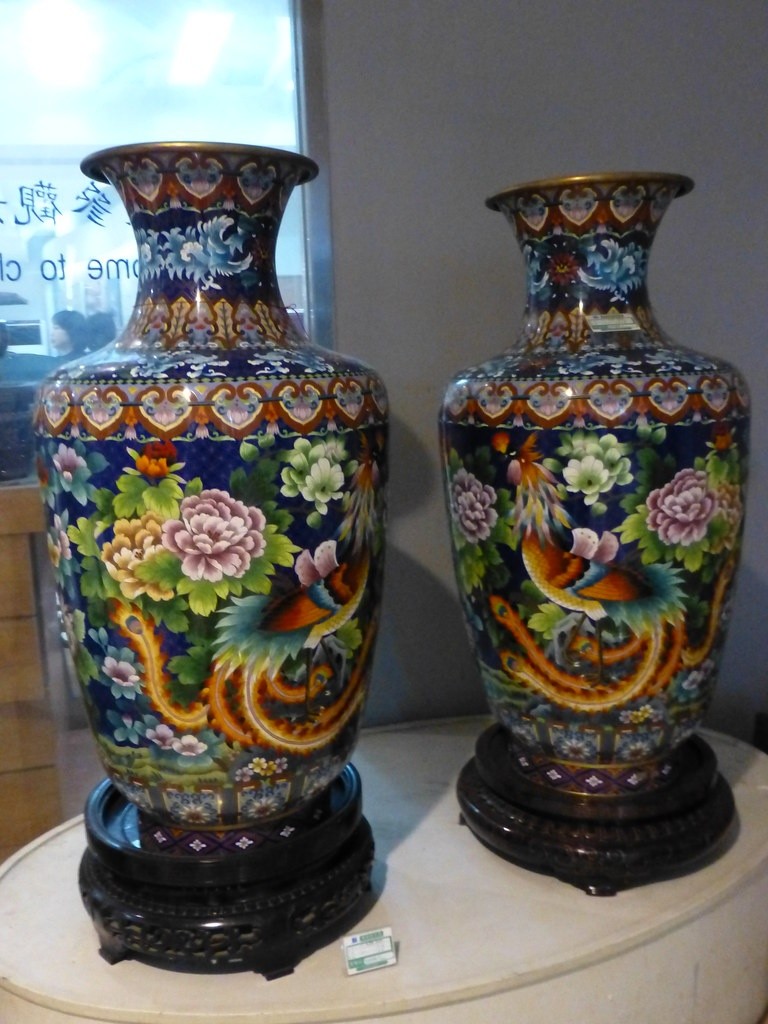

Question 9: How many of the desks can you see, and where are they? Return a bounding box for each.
[0,714,768,1024]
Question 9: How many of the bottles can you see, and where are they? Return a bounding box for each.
[29,141,390,831]
[434,171,754,769]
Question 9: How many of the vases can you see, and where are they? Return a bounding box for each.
[30,142,391,834]
[435,171,755,800]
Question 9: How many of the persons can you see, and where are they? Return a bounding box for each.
[51,310,116,360]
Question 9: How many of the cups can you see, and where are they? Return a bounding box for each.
[0,379,38,480]
